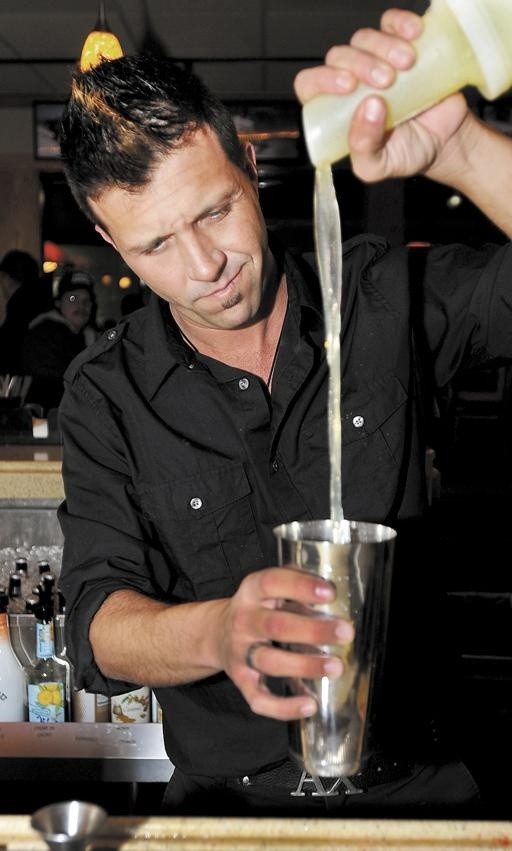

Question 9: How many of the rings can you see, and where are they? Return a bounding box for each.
[245,641,273,668]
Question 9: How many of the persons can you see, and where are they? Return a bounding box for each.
[57,8,512,819]
[0,250,96,411]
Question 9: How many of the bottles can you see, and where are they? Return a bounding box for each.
[299,1,512,168]
[0,556,151,725]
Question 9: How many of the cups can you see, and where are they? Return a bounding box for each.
[255,518,398,776]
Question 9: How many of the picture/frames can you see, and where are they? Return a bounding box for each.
[32,97,68,162]
